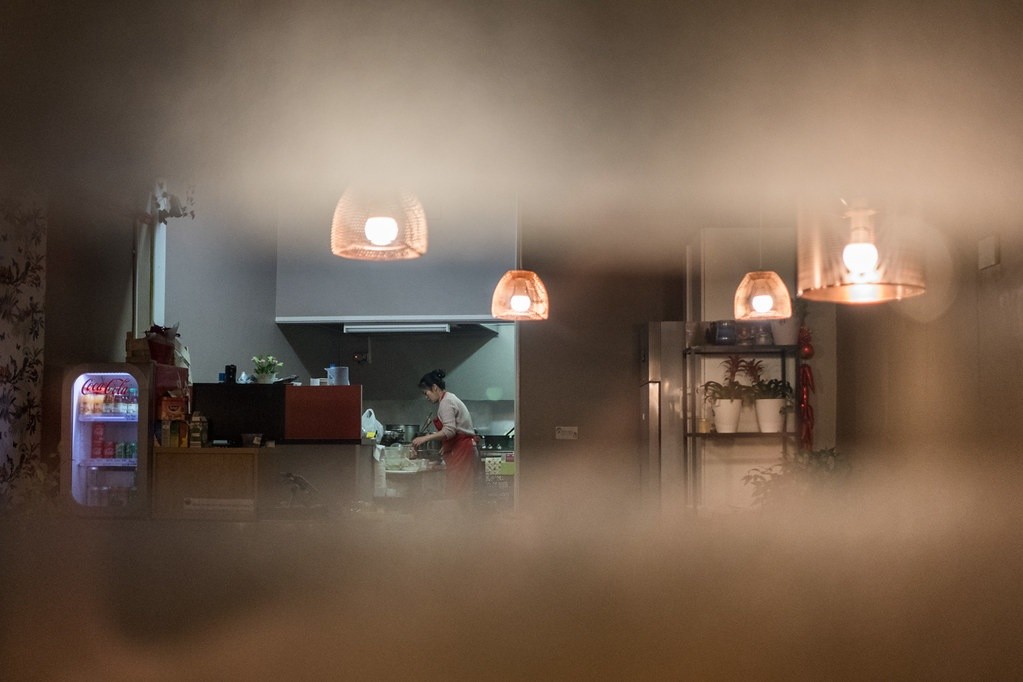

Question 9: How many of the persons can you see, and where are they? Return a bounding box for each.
[412,369,482,499]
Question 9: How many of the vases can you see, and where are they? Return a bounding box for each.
[257,368,277,384]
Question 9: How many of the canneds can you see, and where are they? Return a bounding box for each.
[92,422,136,459]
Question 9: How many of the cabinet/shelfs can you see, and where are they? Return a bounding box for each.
[683,341,810,512]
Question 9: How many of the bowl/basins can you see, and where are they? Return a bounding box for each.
[240,433,264,448]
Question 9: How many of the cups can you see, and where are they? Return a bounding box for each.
[218,364,236,384]
[309,379,320,386]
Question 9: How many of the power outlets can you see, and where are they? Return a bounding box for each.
[353,352,364,361]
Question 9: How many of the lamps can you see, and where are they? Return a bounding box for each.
[732,200,792,320]
[331,172,428,262]
[343,323,451,334]
[791,189,936,305]
[491,198,549,321]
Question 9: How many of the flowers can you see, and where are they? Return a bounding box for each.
[251,353,280,372]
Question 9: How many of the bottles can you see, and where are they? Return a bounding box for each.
[706,320,775,346]
[80,385,139,416]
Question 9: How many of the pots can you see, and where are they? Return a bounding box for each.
[482,435,509,449]
[385,423,420,444]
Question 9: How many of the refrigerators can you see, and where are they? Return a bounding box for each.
[637,320,685,537]
[60,360,187,520]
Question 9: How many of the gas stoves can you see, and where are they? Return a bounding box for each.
[479,444,514,462]
